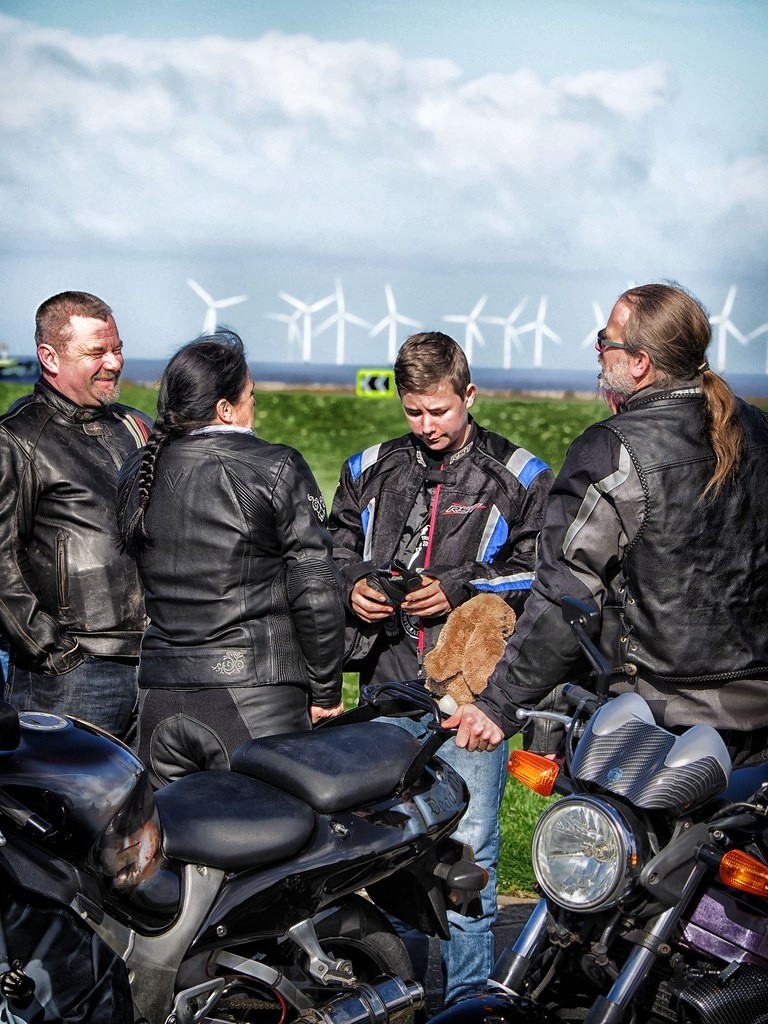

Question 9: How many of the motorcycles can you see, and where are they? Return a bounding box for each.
[0,648,491,1024]
[438,557,768,1023]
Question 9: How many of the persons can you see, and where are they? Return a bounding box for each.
[0,288,167,752]
[441,282,767,773]
[330,327,555,1006]
[116,329,347,792]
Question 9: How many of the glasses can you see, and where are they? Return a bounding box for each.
[596,328,626,350]
